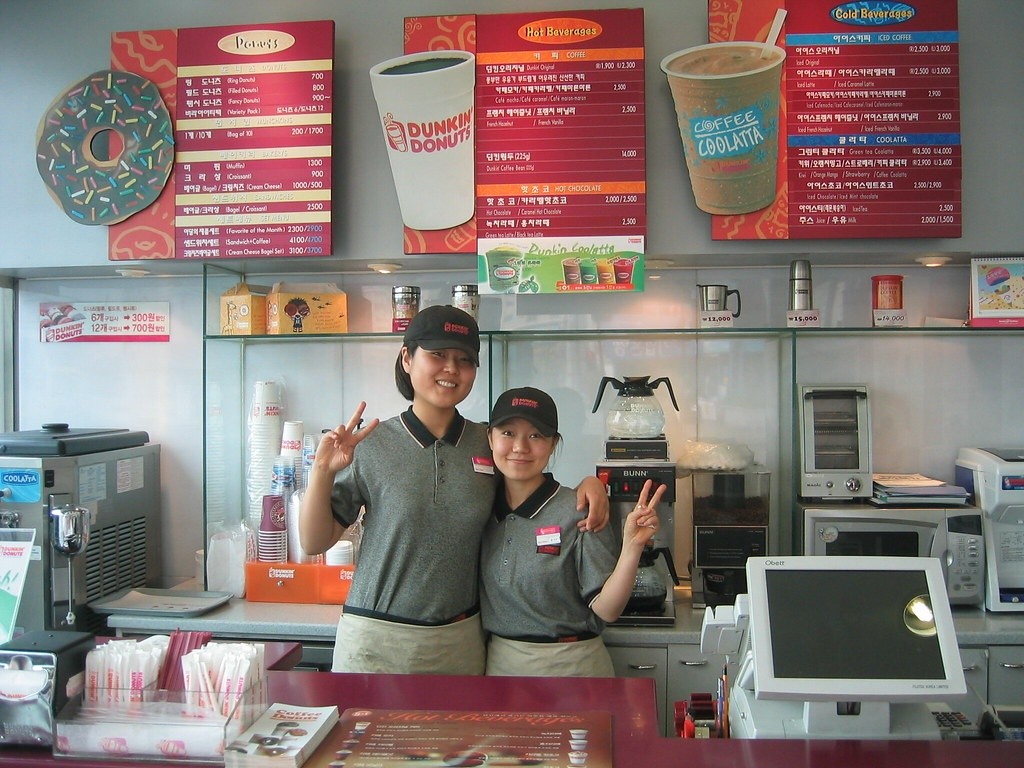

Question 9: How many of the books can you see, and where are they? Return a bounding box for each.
[872,484,968,504]
[223,702,339,768]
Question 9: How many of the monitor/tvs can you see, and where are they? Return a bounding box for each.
[746,555,967,703]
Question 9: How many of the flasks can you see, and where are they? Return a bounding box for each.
[788,260,813,310]
[697,284,741,319]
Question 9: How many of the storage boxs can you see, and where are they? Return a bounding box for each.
[54,672,271,762]
[219,277,350,338]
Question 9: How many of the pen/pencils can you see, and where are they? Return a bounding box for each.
[717,663,730,739]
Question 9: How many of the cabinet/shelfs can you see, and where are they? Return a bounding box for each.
[604,644,742,732]
[928,646,1023,722]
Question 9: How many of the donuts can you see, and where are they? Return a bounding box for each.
[33,71,174,225]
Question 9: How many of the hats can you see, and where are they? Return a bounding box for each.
[486,387,558,438]
[403,305,480,367]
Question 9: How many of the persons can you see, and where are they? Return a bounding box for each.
[486,386,667,677]
[299,305,610,676]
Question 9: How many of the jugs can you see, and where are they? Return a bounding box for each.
[592,376,680,439]
[626,545,679,610]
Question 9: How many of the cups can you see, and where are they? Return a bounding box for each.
[327,722,371,768]
[451,284,480,325]
[660,41,786,214]
[613,263,633,284]
[567,729,588,768]
[561,258,581,287]
[871,275,903,325]
[579,263,597,285]
[486,253,521,291]
[247,381,353,565]
[368,49,476,230]
[597,264,615,285]
[391,285,421,332]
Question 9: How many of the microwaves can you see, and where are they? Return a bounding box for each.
[794,504,985,610]
